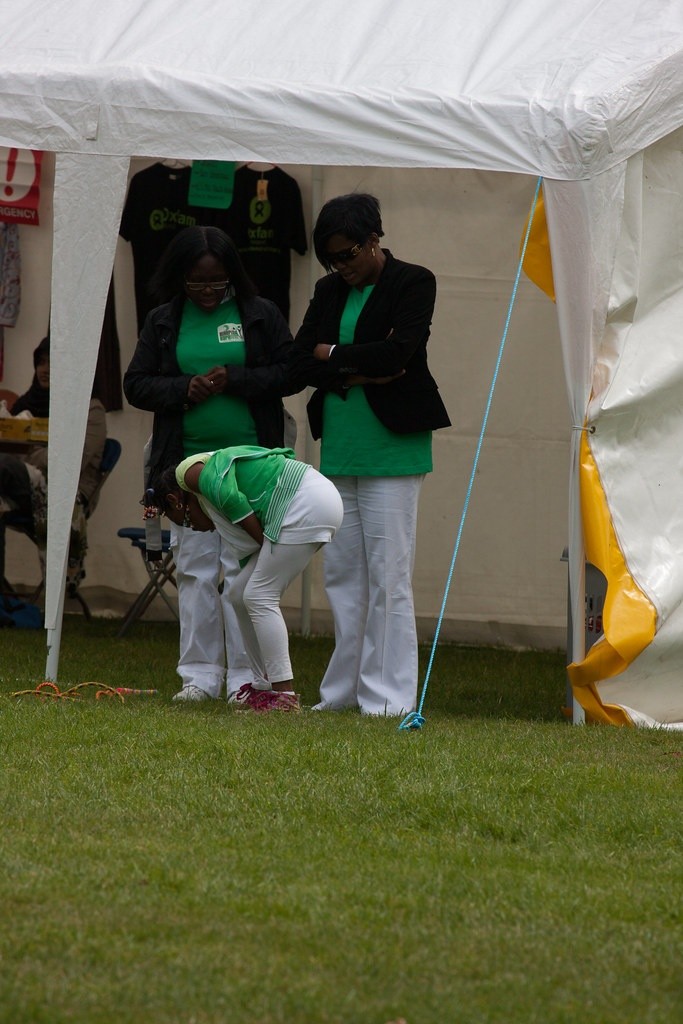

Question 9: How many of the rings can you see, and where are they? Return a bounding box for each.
[211,380,216,386]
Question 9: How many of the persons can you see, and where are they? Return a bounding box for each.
[155,445,346,713]
[0,336,108,599]
[293,193,452,718]
[122,226,308,702]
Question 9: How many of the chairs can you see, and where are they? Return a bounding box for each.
[115,412,297,641]
[0,438,121,619]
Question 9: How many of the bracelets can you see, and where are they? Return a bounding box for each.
[329,344,336,357]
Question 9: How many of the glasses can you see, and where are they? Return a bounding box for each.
[325,240,366,261]
[184,277,226,290]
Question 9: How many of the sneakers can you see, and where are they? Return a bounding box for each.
[172,686,209,701]
[236,682,301,714]
[227,691,245,703]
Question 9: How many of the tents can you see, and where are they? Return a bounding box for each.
[0,0,682,729]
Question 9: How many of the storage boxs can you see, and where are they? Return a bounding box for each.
[1,418,50,440]
